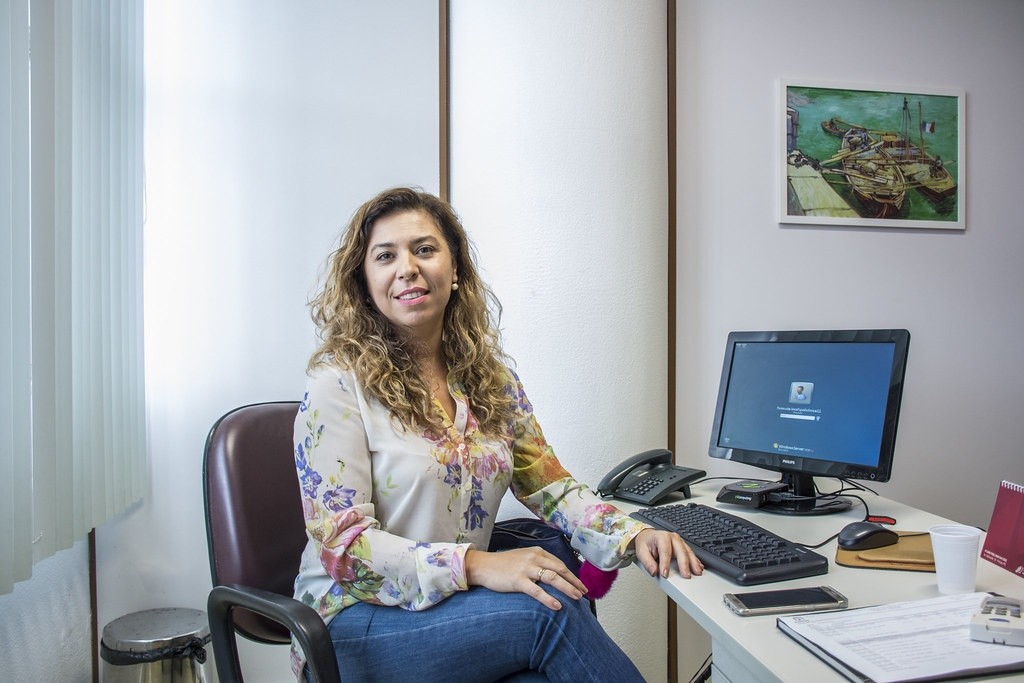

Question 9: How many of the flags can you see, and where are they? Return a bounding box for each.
[921,121,936,133]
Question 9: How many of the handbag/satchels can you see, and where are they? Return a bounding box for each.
[487,518,581,575]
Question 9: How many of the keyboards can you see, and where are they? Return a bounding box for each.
[628,502,829,587]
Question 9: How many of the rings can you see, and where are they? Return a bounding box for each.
[538,567,548,582]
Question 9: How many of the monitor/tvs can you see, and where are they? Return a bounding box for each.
[708,328,911,517]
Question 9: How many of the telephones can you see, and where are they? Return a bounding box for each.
[597,448,707,506]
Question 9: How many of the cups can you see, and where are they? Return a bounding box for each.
[928,524,982,595]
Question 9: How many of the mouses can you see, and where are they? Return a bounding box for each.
[838,522,900,550]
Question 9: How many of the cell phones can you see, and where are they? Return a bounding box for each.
[723,585,848,615]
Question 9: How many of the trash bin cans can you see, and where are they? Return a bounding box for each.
[100,605,210,682]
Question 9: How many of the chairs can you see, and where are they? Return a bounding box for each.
[204,399,341,683]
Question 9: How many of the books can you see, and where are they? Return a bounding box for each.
[777,591,1024,683]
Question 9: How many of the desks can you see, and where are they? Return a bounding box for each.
[600,478,1024,683]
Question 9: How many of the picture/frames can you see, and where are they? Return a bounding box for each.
[771,78,969,231]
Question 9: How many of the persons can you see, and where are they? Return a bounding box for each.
[288,188,706,683]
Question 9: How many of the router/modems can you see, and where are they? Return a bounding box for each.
[716,481,790,507]
